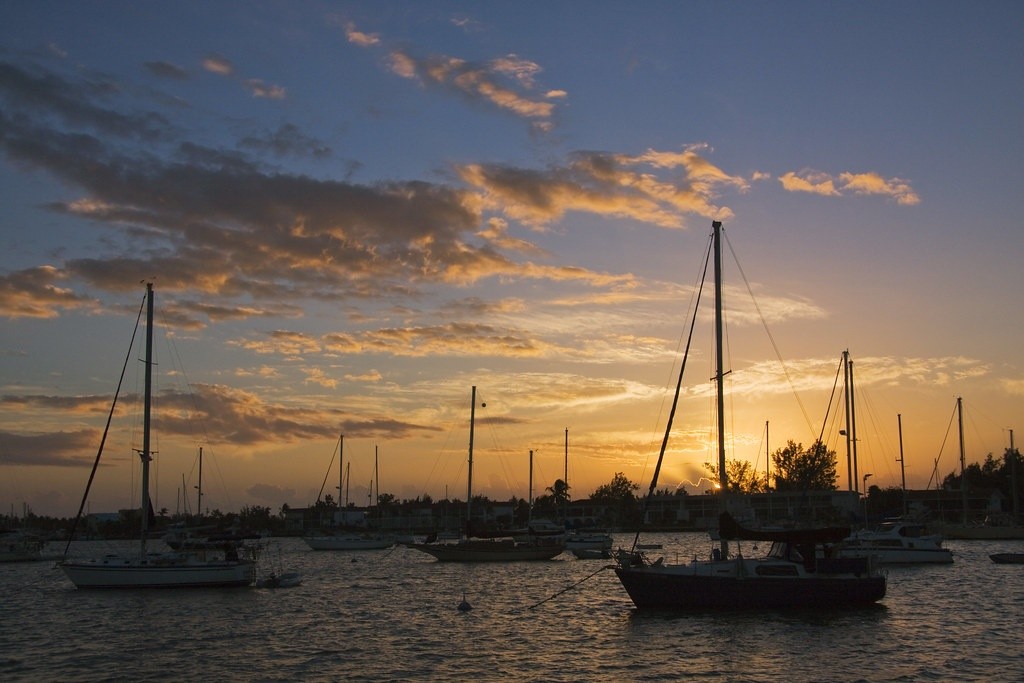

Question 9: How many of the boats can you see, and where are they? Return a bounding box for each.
[989,553,1024,565]
[941,511,1024,541]
[634,543,664,550]
[0,502,50,563]
[716,509,853,545]
[826,518,953,563]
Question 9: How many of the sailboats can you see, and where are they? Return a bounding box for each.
[303,433,398,550]
[565,429,614,559]
[58,283,260,591]
[613,217,891,613]
[511,447,571,542]
[168,472,218,536]
[271,569,302,587]
[407,387,564,562]
[365,443,428,544]
[314,460,376,541]
[164,447,244,551]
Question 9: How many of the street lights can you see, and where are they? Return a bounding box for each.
[862,474,872,498]
